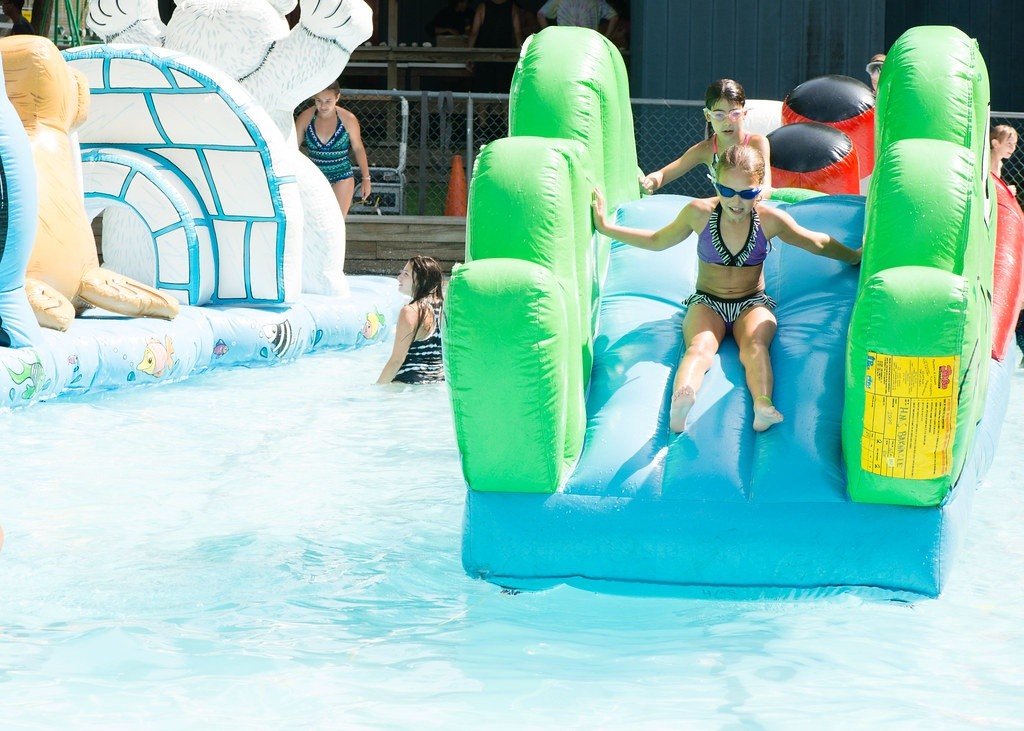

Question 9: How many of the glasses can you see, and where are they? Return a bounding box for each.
[705,108,746,121]
[715,182,762,200]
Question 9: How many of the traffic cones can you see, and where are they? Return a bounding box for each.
[443,155,467,217]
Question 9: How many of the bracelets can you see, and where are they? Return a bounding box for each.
[362,175,371,180]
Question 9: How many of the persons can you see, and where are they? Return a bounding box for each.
[376,255,445,386]
[537,0,618,41]
[591,145,862,432]
[467,0,523,143]
[297,81,371,221]
[990,124,1018,197]
[424,0,475,48]
[867,54,886,91]
[637,79,772,200]
